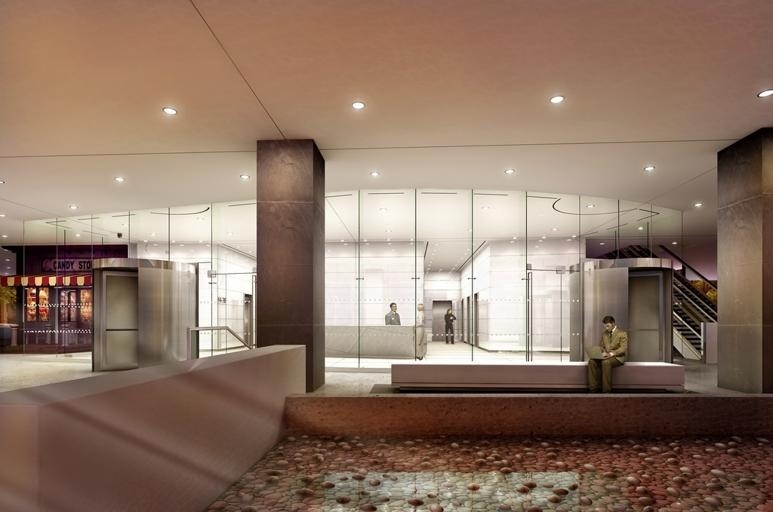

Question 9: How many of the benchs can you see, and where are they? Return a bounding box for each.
[390,360,685,393]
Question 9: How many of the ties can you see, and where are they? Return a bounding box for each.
[610,330,612,344]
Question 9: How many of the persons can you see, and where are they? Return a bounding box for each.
[587,316,628,393]
[385,303,400,325]
[416,304,426,360]
[444,308,456,344]
